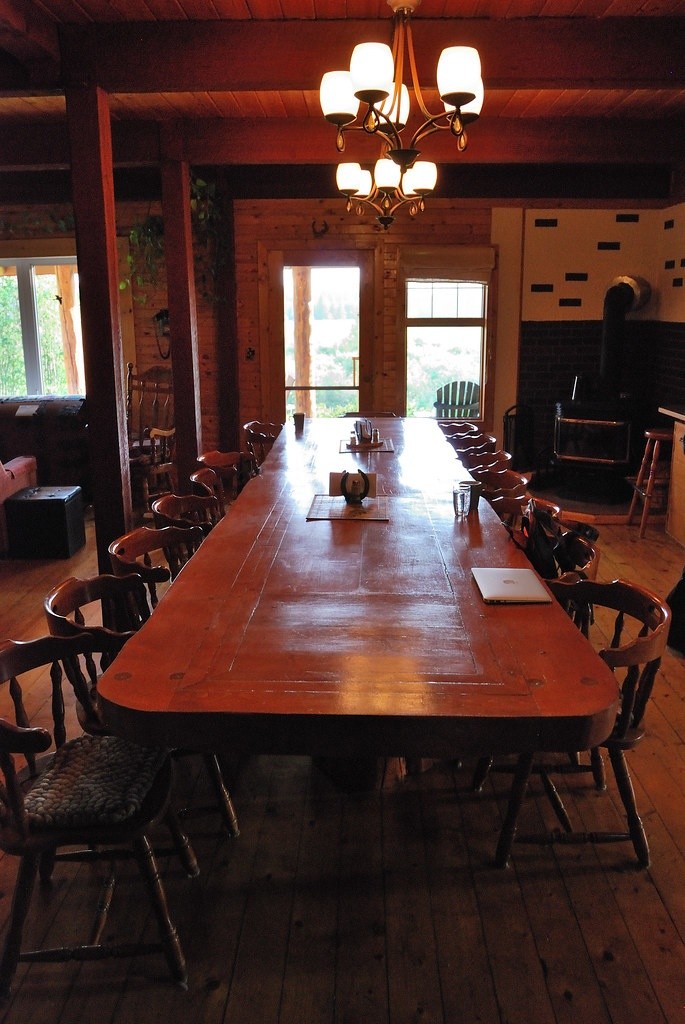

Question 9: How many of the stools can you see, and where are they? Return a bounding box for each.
[4,485,86,560]
[626,428,674,540]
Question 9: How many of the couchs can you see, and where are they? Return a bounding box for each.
[0,455,38,555]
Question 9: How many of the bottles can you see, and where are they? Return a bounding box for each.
[351,421,379,446]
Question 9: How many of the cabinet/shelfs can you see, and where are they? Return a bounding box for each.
[553,414,631,464]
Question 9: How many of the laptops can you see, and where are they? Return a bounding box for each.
[471,567,552,605]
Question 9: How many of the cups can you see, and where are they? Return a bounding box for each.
[453,484,471,516]
[294,413,305,428]
[459,480,481,510]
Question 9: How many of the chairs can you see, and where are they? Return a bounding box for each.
[473,578,673,872]
[126,362,175,514]
[196,450,255,517]
[108,525,203,631]
[242,421,284,467]
[44,572,242,841]
[440,422,603,632]
[434,381,480,417]
[0,633,201,995]
[189,467,226,532]
[151,494,223,582]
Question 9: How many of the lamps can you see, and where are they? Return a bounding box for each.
[336,137,438,230]
[319,0,484,173]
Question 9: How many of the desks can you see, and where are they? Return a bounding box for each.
[96,417,621,792]
